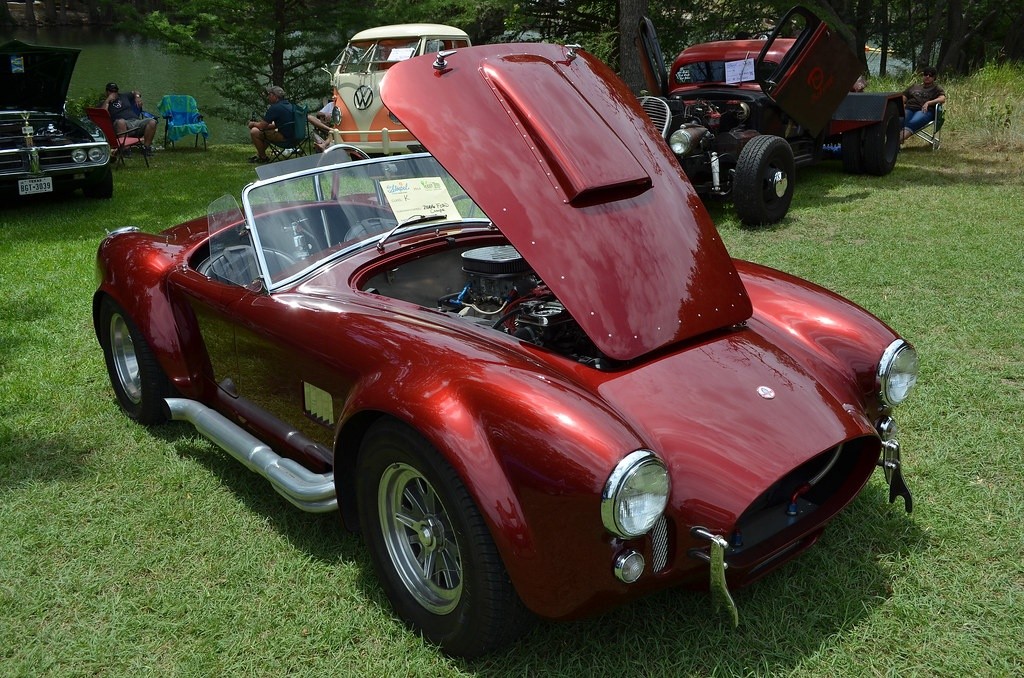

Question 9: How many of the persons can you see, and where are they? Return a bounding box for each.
[100,82,156,158]
[896,67,945,142]
[306,96,334,151]
[247,86,296,163]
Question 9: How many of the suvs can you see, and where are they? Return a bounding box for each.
[623,5,907,229]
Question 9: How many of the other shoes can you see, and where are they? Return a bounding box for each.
[138,146,151,156]
[249,155,270,164]
[124,149,131,157]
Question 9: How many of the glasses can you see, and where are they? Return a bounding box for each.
[924,72,933,77]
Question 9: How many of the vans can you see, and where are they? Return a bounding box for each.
[326,22,473,155]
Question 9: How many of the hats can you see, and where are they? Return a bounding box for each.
[265,85,285,97]
[106,83,119,93]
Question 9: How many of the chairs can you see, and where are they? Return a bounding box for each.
[344,217,398,241]
[262,104,310,165]
[85,94,208,171]
[913,102,945,151]
[195,245,296,287]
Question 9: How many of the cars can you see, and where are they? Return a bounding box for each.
[89,44,920,658]
[0,38,113,205]
